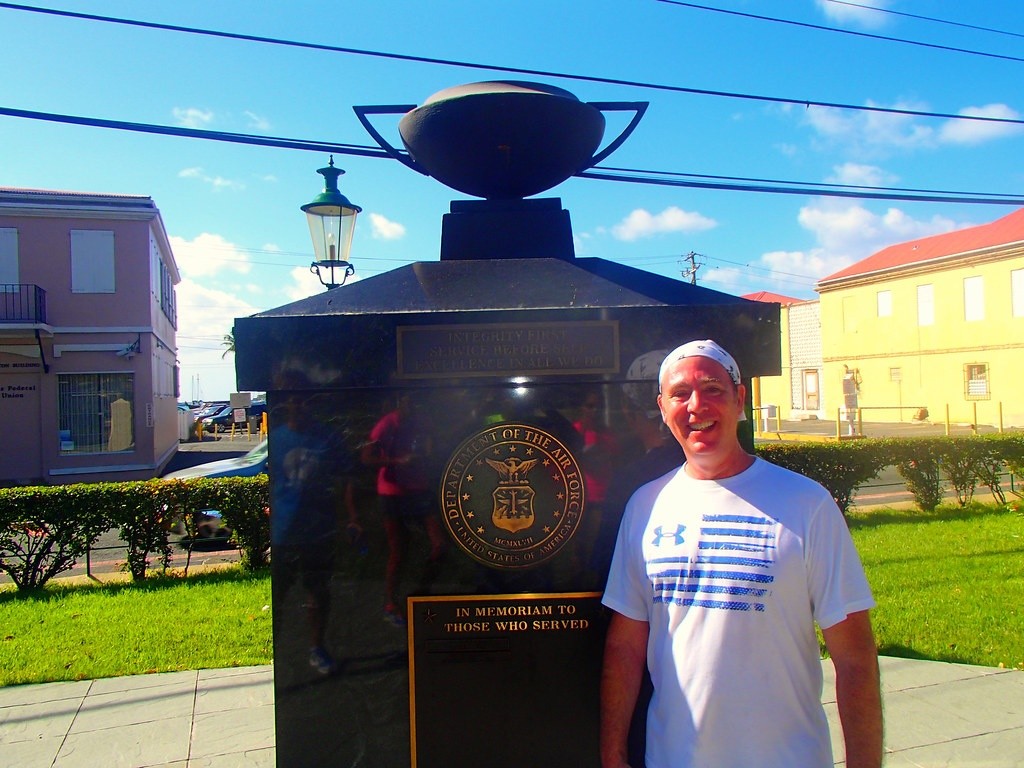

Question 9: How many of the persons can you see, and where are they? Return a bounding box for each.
[602,341,884,768]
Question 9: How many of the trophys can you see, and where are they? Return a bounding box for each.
[352,80,651,261]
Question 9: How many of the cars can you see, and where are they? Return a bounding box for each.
[177,401,229,423]
[162,439,267,550]
[201,401,267,433]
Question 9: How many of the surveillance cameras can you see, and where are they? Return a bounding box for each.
[115,348,130,357]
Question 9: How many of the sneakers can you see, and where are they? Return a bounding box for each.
[384,605,407,628]
[310,648,337,674]
